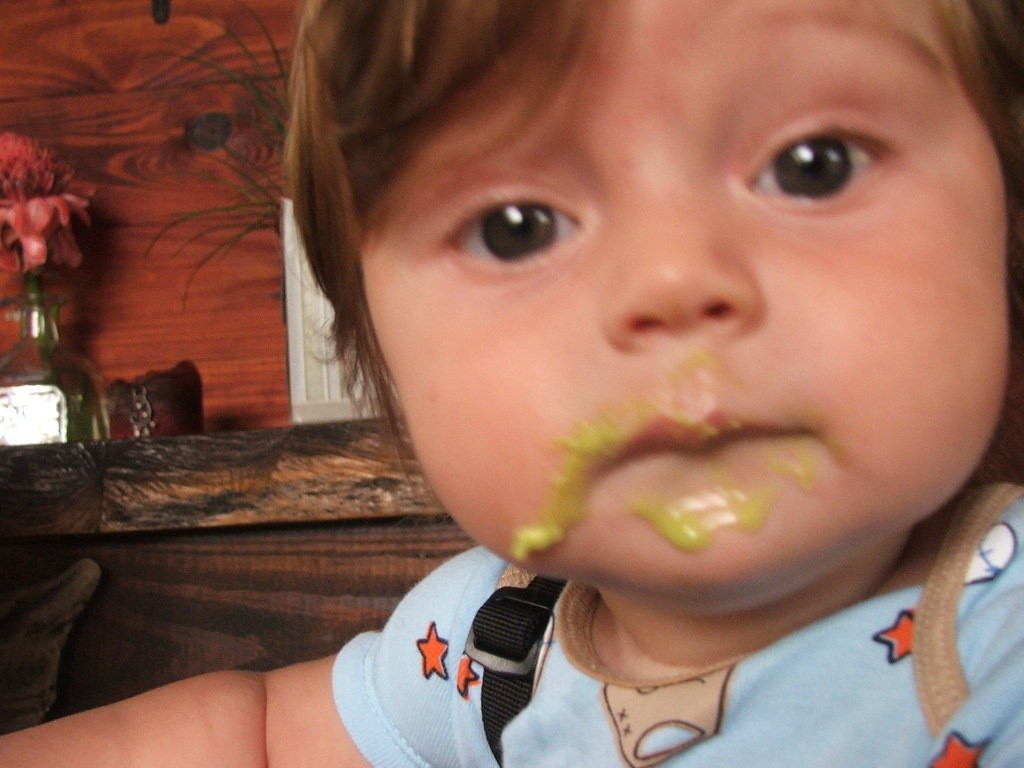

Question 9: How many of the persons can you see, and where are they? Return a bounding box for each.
[0,0,1024,768]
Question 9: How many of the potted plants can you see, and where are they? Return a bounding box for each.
[144,3,401,423]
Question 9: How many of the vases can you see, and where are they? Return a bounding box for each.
[0,294,111,448]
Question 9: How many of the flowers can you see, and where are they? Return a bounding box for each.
[0,131,115,435]
